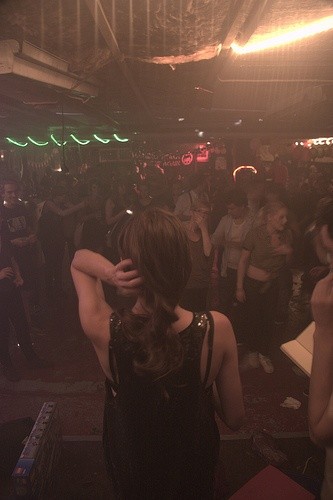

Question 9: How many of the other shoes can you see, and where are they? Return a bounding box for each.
[256,352,274,375]
[248,350,261,370]
[274,314,291,325]
[2,364,21,382]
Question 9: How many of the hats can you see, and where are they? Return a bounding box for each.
[189,172,209,190]
[314,196,333,219]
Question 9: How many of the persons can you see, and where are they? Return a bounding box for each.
[72,206,248,500]
[0,166,333,383]
[307,202,333,500]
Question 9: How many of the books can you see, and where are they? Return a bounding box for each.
[279,320,315,379]
